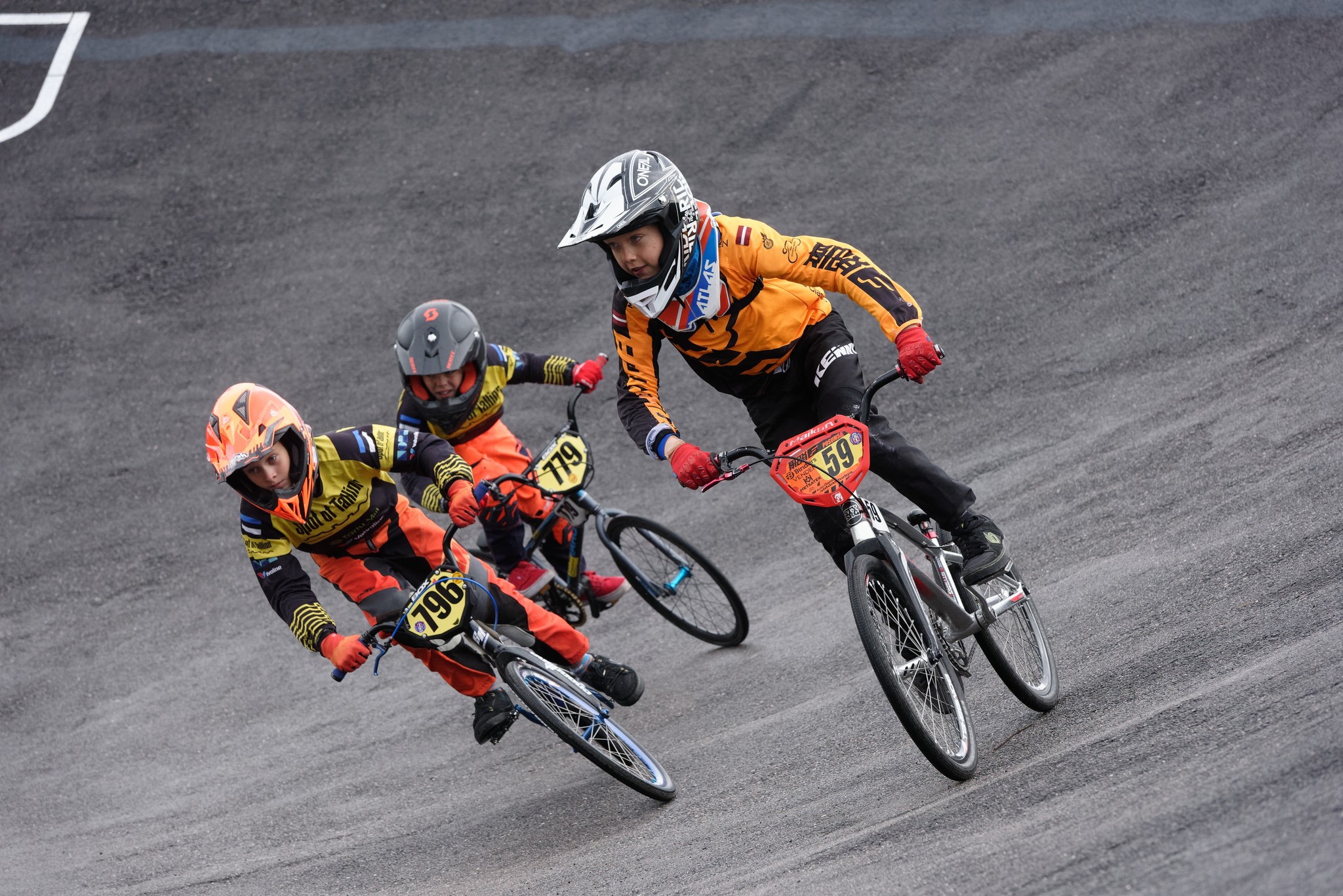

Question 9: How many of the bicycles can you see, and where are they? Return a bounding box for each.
[704,343,1058,781]
[329,486,676,804]
[459,351,751,652]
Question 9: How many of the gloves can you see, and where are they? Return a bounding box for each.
[572,360,604,394]
[670,443,720,491]
[448,489,490,516]
[321,632,374,673]
[447,479,479,528]
[895,327,942,384]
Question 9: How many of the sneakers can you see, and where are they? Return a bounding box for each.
[951,513,1011,586]
[904,652,964,714]
[506,561,556,598]
[471,688,516,745]
[581,653,644,707]
[579,571,632,606]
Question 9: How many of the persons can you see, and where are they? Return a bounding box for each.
[204,380,646,745]
[555,150,1009,716]
[391,297,636,603]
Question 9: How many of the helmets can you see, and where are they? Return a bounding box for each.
[394,299,485,435]
[557,149,698,319]
[205,382,319,525]
